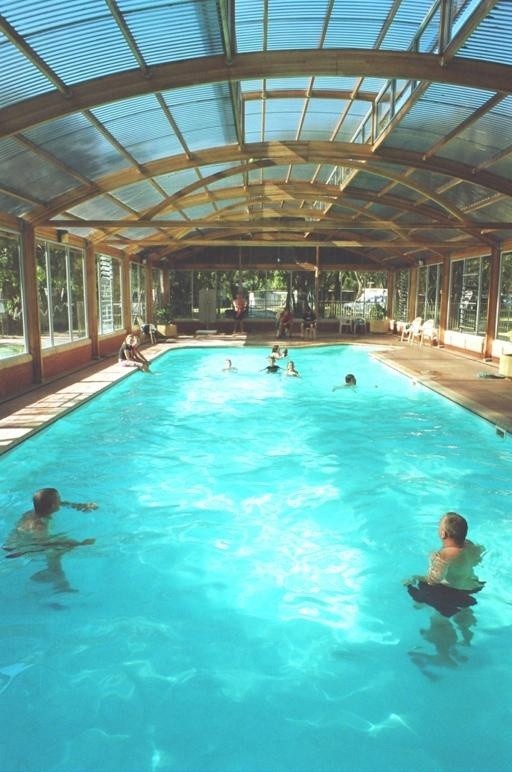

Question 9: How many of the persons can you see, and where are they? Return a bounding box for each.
[300,306,318,340]
[259,357,288,376]
[132,335,152,369]
[220,358,239,373]
[283,360,299,376]
[3,485,99,596]
[401,512,487,670]
[232,292,250,336]
[140,324,157,346]
[118,333,150,372]
[330,373,358,392]
[269,344,288,359]
[279,308,294,339]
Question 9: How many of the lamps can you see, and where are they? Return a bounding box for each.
[57,230,69,243]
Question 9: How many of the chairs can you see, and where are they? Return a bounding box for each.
[336,315,366,336]
[401,317,440,347]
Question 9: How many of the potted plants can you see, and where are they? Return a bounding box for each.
[155,300,178,337]
[369,303,389,334]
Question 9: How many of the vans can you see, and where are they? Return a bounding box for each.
[277,290,316,312]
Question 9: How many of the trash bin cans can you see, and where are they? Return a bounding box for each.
[499,354,512,378]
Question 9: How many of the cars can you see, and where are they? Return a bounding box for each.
[343,290,388,319]
[129,289,147,317]
[458,290,512,314]
